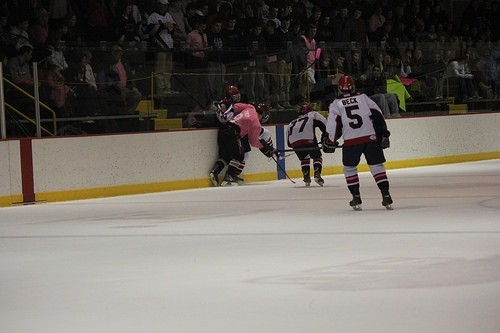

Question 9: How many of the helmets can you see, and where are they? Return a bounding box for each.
[338,75,356,95]
[227,86,241,102]
[301,103,312,114]
[256,103,270,123]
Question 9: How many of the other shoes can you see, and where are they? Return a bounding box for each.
[160,87,180,95]
[269,101,285,110]
[282,102,295,109]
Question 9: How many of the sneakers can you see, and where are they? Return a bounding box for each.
[232,174,244,185]
[349,193,363,211]
[314,171,325,186]
[210,166,225,187]
[221,172,239,187]
[382,194,394,210]
[302,174,311,185]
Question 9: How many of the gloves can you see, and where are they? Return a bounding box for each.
[259,145,274,158]
[382,131,390,149]
[321,133,330,144]
[323,138,339,154]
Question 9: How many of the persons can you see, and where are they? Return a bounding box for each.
[322,74,393,206]
[209,85,273,186]
[0,0,500,119]
[286,102,339,185]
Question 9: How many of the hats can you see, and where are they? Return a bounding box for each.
[156,0,169,5]
[195,10,204,17]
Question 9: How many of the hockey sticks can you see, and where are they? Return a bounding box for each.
[275,145,341,152]
[278,141,322,160]
[274,151,290,160]
[272,156,295,184]
